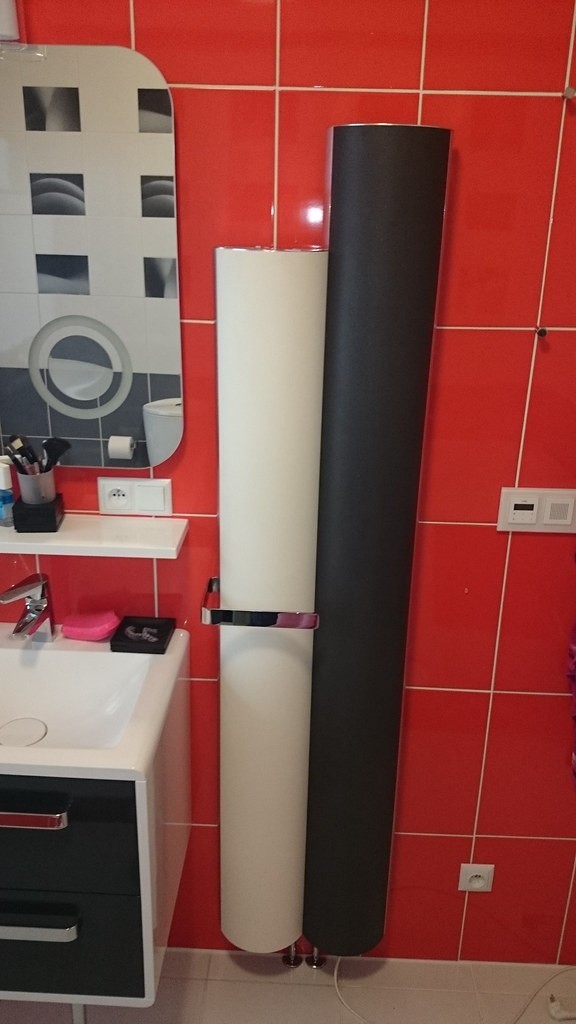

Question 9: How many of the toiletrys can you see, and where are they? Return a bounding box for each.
[0,464,20,529]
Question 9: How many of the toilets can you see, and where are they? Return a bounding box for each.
[142,397,184,467]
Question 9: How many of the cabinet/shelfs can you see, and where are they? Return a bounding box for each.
[0,631,190,1007]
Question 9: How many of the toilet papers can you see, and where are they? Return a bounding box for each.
[107,434,134,462]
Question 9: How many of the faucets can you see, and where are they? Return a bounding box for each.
[1,574,59,644]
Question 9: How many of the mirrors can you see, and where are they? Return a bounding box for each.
[0,41,184,470]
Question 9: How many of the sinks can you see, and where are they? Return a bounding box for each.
[1,618,192,786]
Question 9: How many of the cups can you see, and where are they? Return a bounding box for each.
[16,467,54,505]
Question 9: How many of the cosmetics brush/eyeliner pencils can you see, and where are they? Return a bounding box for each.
[3,433,71,474]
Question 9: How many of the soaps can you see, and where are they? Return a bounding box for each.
[61,612,123,644]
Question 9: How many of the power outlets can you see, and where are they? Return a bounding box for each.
[458,863,495,893]
[497,487,574,533]
[96,475,135,514]
